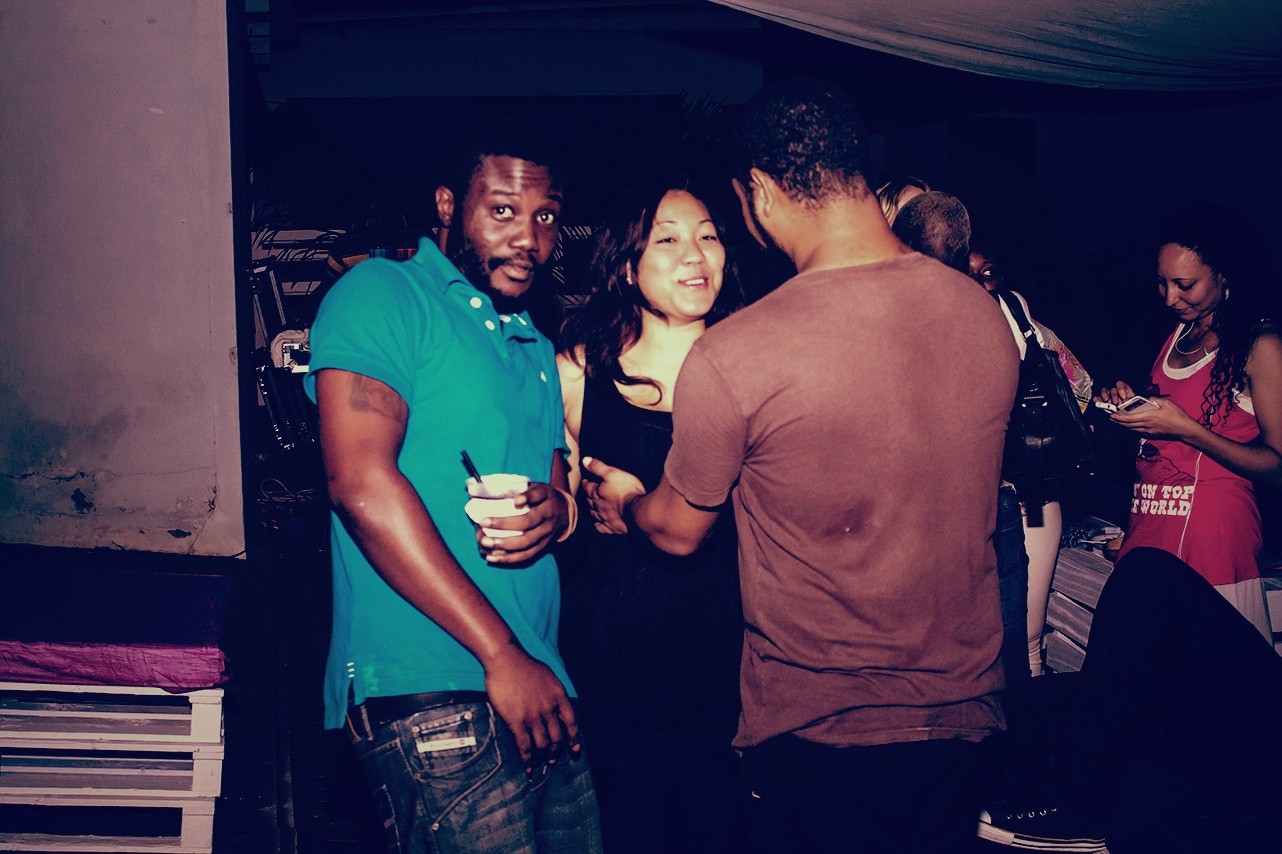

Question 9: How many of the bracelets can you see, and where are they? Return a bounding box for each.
[554,484,577,541]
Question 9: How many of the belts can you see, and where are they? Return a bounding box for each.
[343,690,490,739]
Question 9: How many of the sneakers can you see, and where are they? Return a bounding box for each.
[977,794,1107,852]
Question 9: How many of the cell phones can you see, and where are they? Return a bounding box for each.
[1095,396,1158,414]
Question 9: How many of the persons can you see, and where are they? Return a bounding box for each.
[306,121,603,854]
[542,78,1282,854]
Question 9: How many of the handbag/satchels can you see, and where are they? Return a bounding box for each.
[995,289,1097,508]
